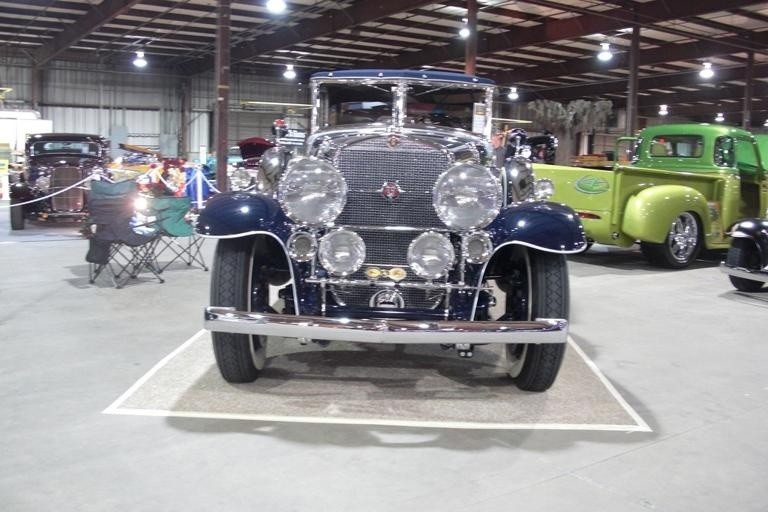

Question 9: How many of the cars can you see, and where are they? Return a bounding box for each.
[8,132,115,230]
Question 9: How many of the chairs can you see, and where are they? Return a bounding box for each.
[79,176,208,289]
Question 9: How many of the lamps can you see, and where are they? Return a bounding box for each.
[507,86,519,100]
[699,62,714,78]
[597,40,612,61]
[658,104,669,116]
[132,52,147,67]
[715,112,724,122]
[283,65,296,80]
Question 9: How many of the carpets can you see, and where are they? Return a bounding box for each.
[99,327,655,433]
